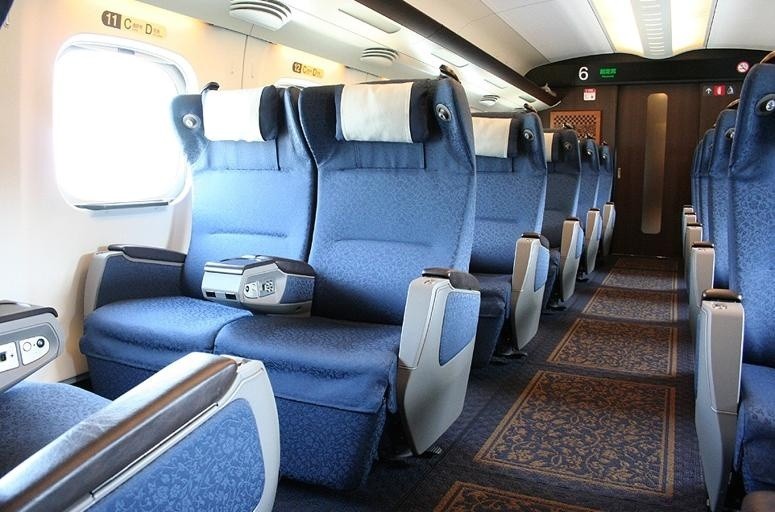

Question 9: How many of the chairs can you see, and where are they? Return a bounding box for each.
[469,102,553,359]
[596,136,617,264]
[541,122,586,312]
[682,63,775,512]
[79,63,481,487]
[576,132,603,282]
[2,294,283,511]
